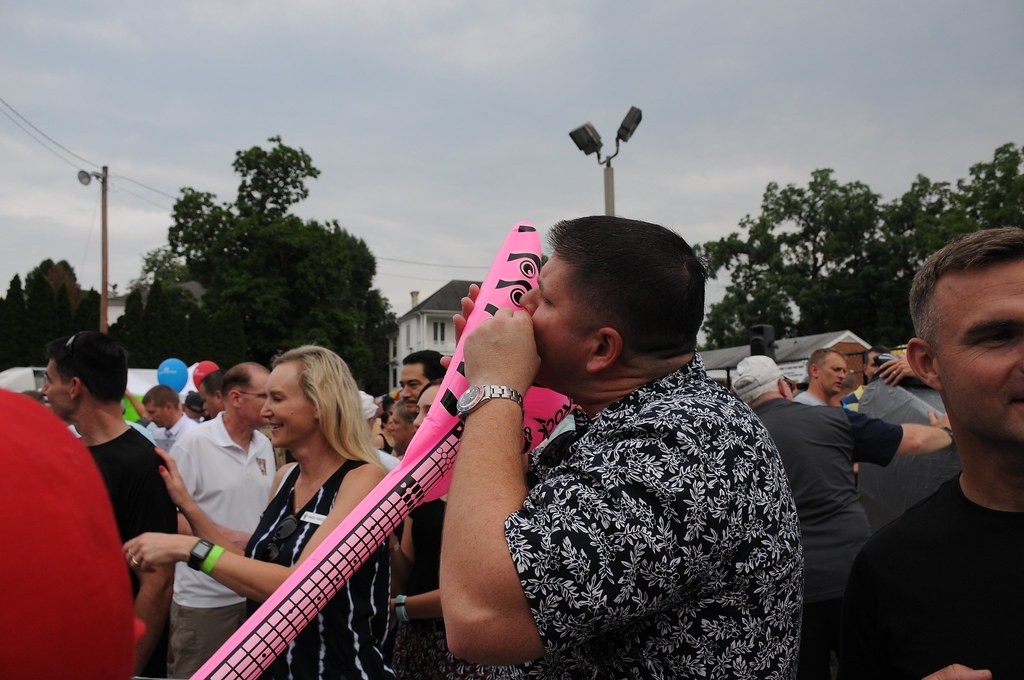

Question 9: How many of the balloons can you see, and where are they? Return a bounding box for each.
[158,357,188,394]
[193,360,218,392]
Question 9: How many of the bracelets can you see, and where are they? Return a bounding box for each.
[396,595,409,622]
[201,542,225,573]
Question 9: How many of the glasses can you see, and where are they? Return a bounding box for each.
[67,330,87,362]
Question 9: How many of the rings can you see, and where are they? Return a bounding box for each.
[128,548,134,557]
[131,556,140,566]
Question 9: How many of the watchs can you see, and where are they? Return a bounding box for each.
[187,537,216,570]
[456,382,525,425]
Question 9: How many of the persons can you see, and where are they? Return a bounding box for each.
[437,214,807,680]
[833,223,1024,680]
[731,343,962,680]
[19,328,449,680]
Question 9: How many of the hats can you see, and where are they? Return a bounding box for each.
[732,355,797,397]
